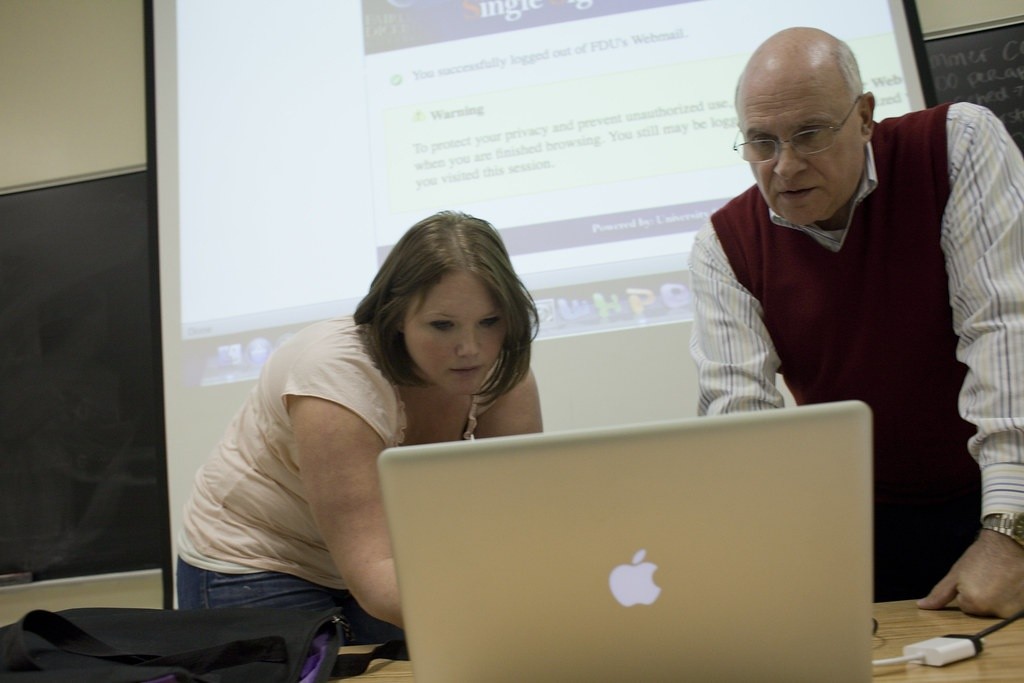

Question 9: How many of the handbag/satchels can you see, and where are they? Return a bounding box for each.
[0,606,408,683]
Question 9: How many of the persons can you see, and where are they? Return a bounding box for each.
[689,27,1024,620]
[176,211,542,646]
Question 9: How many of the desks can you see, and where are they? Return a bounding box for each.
[329,600,1024,683]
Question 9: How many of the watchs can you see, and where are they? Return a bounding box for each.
[982,512,1024,547]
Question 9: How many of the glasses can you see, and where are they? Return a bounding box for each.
[733,95,862,163]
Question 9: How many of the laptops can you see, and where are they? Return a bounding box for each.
[378,399,873,683]
